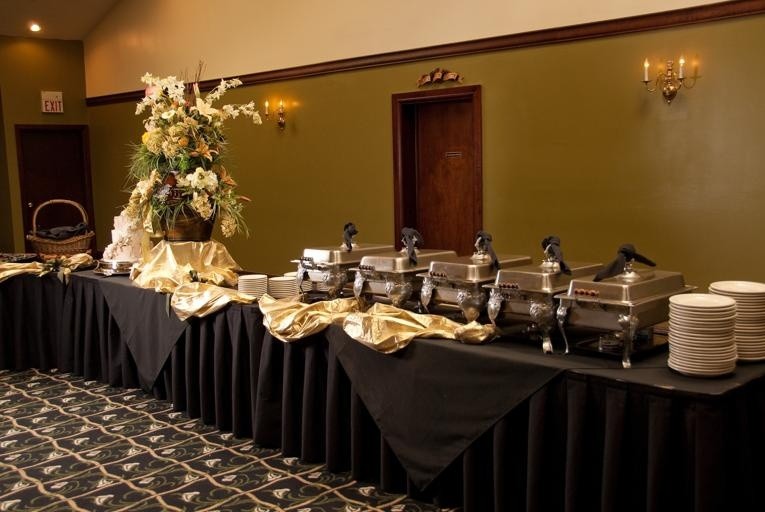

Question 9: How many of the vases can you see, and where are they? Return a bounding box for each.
[158,189,218,241]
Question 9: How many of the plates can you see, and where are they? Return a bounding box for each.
[667,280,765,378]
[238,271,332,300]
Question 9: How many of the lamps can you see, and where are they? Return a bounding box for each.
[264,99,288,133]
[643,57,687,105]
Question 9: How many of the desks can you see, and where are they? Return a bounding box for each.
[0,252,765,512]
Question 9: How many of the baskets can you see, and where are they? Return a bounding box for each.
[26,199,95,256]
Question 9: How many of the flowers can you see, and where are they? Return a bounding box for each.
[116,60,263,240]
